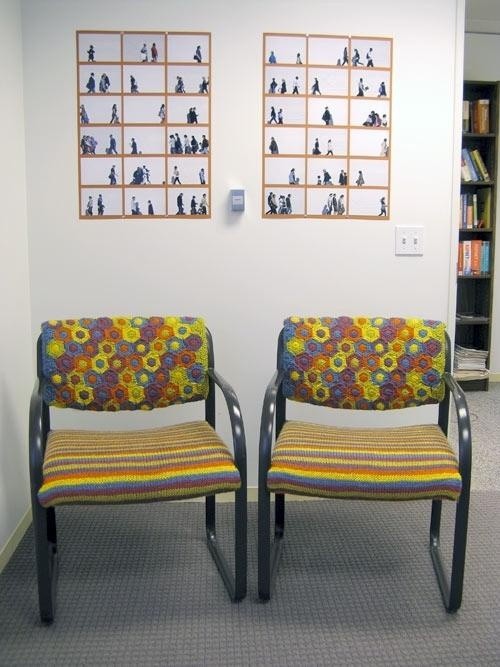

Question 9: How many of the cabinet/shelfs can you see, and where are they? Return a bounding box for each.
[453,80,500,392]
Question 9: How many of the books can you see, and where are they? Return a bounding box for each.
[457,239,490,275]
[463,99,490,133]
[457,312,489,322]
[454,342,489,371]
[460,147,491,182]
[460,187,491,228]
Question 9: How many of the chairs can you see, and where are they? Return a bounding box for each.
[27,316,247,625]
[258,315,472,615]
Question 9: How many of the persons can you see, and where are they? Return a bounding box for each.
[77,39,212,217]
[264,46,391,217]
